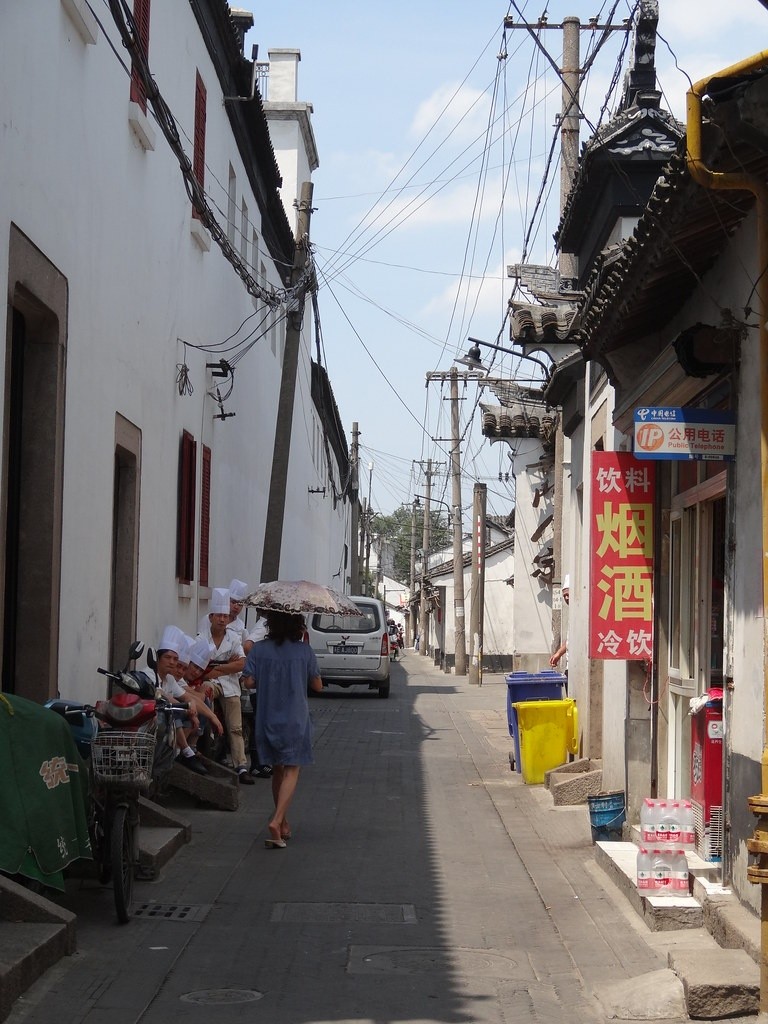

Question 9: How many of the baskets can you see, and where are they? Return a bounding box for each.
[91,729,156,787]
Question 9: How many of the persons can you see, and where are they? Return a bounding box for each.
[386,620,403,652]
[141,625,224,774]
[550,575,569,696]
[199,580,273,785]
[243,610,323,848]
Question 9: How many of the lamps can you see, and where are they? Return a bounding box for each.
[452,336,550,382]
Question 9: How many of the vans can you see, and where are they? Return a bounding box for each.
[301,595,391,699]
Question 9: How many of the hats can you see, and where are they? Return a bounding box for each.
[178,635,196,664]
[228,578,249,602]
[209,587,230,616]
[158,625,185,660]
[190,639,215,671]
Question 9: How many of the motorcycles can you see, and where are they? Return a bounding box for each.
[389,631,405,662]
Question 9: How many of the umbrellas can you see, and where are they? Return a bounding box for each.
[236,581,364,616]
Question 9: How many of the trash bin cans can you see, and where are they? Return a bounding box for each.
[505,670,568,774]
[511,698,580,785]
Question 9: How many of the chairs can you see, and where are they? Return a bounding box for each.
[359,618,374,628]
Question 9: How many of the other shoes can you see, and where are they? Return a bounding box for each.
[238,771,256,786]
[201,731,216,747]
[176,750,208,774]
[250,758,274,776]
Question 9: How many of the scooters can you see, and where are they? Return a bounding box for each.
[62,639,198,923]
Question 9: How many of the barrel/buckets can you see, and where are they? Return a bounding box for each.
[586,789,627,845]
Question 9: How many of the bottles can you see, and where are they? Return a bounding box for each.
[636,798,698,896]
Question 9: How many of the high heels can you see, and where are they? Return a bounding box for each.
[281,829,290,839]
[265,838,286,850]
